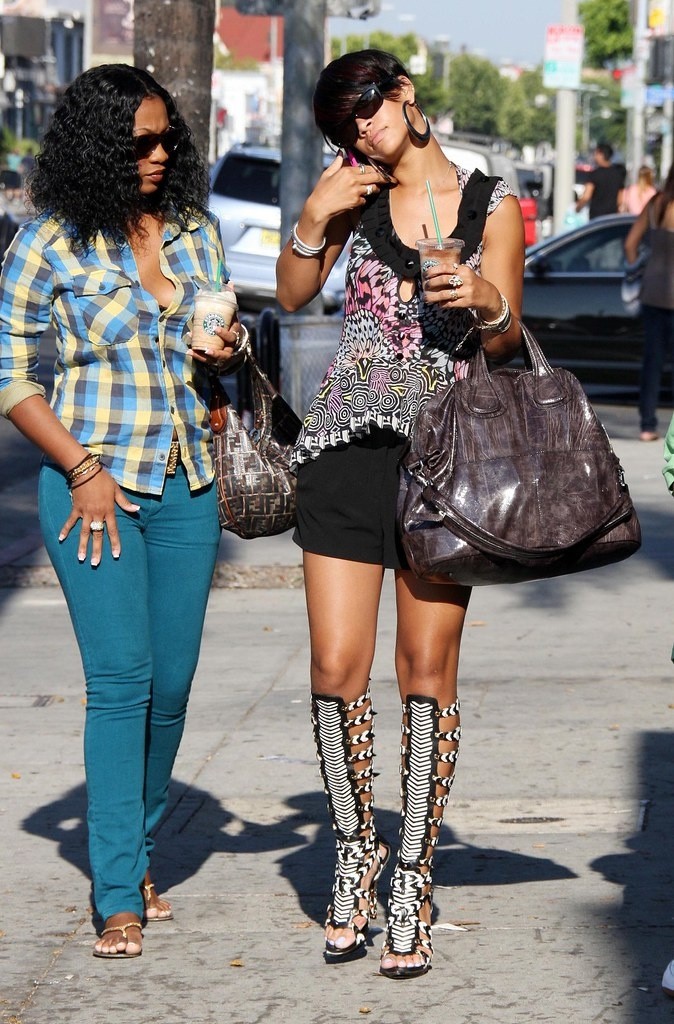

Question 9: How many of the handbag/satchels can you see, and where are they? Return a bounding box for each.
[399,312,643,586]
[201,318,304,541]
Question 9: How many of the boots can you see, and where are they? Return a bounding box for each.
[309,679,390,964]
[378,693,462,980]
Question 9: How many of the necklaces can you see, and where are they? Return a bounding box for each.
[411,161,452,237]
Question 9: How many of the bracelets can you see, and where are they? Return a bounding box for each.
[471,293,512,334]
[234,323,250,352]
[66,453,110,492]
[290,221,327,257]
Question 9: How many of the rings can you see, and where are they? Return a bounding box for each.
[449,275,463,287]
[450,290,458,300]
[90,521,104,531]
[359,164,365,174]
[453,263,458,273]
[216,359,225,367]
[367,186,373,195]
[233,331,240,344]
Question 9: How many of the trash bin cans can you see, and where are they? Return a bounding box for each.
[277,316,345,424]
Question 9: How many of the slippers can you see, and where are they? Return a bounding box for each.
[141,883,173,922]
[93,923,144,958]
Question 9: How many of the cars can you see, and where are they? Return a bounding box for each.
[496,212,674,412]
[431,123,625,250]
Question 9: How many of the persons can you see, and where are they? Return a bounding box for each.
[6,148,36,202]
[275,50,526,979]
[623,162,674,442]
[0,64,244,957]
[624,165,658,215]
[576,144,625,221]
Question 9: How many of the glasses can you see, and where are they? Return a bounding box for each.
[131,126,181,159]
[331,79,384,148]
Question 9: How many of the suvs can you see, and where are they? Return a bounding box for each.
[206,145,352,316]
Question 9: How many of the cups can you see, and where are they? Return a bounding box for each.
[191,284,238,352]
[416,238,465,299]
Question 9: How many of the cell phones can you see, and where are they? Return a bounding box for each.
[345,146,372,168]
